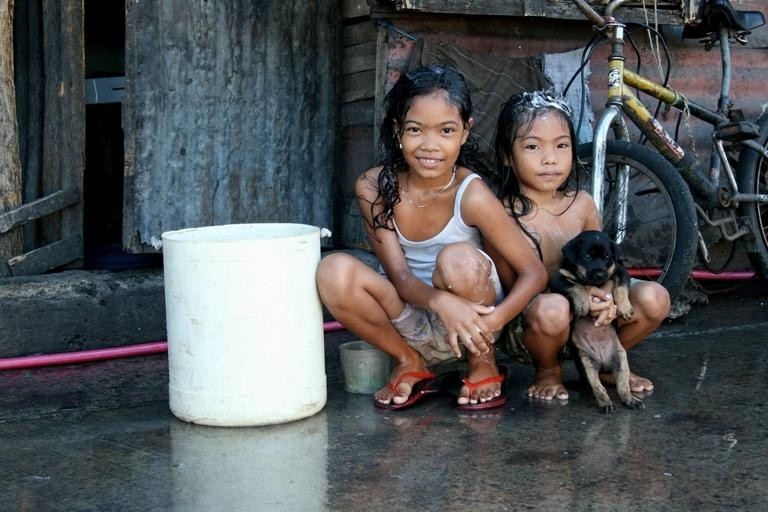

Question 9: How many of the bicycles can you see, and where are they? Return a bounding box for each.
[562,0,768,305]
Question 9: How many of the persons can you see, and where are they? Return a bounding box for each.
[317,64,548,408]
[482,88,670,400]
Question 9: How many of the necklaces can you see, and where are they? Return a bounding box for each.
[401,168,456,207]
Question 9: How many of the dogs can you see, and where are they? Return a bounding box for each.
[548,227,647,416]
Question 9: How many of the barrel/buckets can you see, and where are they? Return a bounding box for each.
[160,223,328,428]
[170,411,328,512]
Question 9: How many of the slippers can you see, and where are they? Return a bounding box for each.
[374,372,444,410]
[456,375,507,410]
